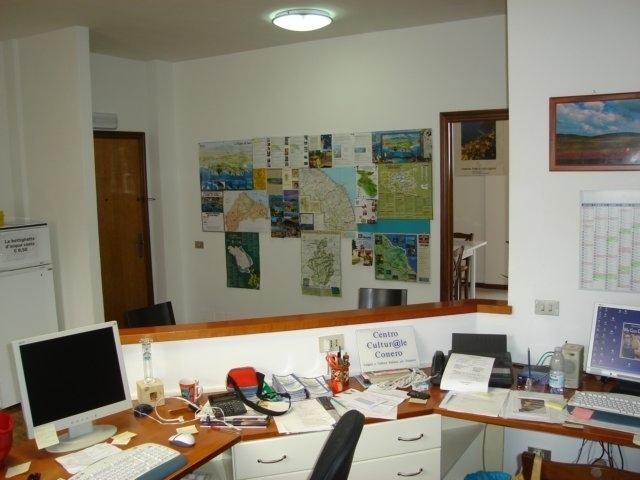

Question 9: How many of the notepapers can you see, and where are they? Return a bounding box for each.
[544,398,568,411]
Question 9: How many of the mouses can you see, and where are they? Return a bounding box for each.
[169,433,196,447]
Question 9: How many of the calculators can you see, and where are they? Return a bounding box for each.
[208,390,247,418]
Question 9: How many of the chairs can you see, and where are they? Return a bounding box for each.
[125,301,176,327]
[453,232,473,299]
[359,288,407,309]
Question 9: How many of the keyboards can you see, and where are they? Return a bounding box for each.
[567,390,640,420]
[67,443,187,480]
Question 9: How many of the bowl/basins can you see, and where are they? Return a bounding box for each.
[0,412,13,467]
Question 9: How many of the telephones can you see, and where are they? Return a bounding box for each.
[430,351,448,385]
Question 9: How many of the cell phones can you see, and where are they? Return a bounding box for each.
[407,388,430,400]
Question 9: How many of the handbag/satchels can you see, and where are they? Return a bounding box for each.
[227,367,258,397]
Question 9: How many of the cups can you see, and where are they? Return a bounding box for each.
[179,377,203,405]
[330,369,350,394]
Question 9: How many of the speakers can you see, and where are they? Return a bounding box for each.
[560,343,584,389]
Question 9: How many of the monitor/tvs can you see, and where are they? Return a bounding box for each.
[13,320,133,454]
[584,303,640,384]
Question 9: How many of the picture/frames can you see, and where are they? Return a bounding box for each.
[548,91,640,172]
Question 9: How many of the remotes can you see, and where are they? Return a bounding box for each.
[317,395,335,409]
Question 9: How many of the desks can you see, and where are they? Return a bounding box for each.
[1,362,640,480]
[453,241,488,299]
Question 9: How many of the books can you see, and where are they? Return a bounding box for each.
[196,398,268,428]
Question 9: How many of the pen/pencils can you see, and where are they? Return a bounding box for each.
[527,348,531,378]
[325,346,350,370]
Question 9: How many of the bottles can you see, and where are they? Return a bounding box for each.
[141,343,154,385]
[261,380,279,400]
[524,378,533,391]
[515,376,525,391]
[547,346,565,395]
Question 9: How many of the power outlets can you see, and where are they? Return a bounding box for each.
[319,335,345,352]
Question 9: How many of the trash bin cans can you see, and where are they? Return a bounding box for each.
[464,470,512,480]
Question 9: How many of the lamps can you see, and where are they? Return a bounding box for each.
[269,8,334,31]
